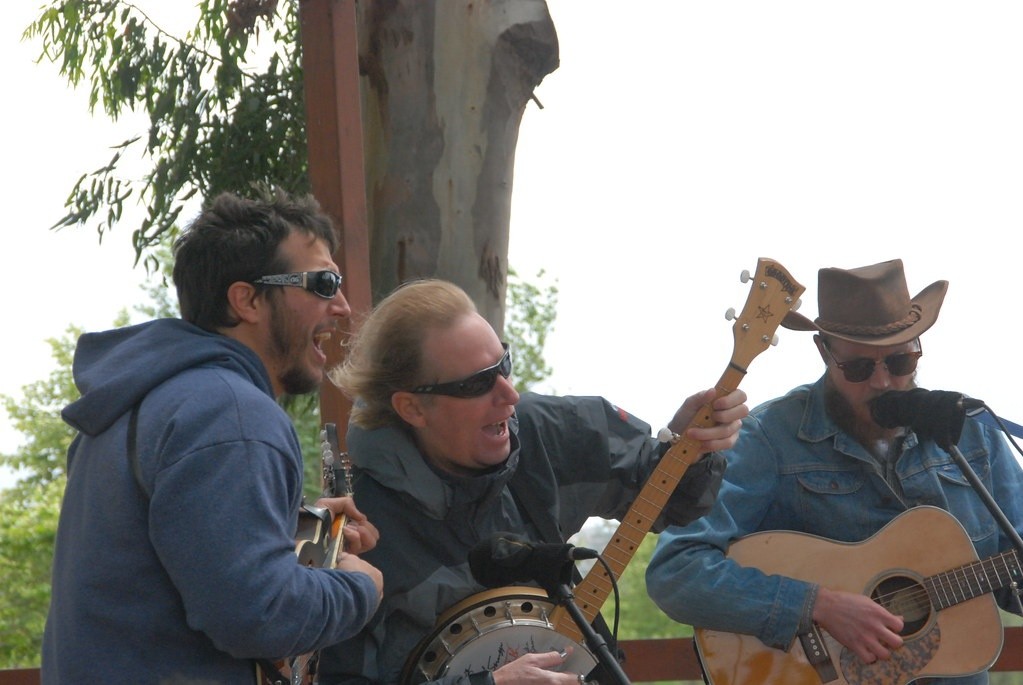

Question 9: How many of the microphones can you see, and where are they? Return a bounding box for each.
[871,390,985,429]
[467,531,598,588]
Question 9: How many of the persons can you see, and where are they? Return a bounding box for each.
[41,181,383,685]
[645,259,1023,685]
[317,279,748,685]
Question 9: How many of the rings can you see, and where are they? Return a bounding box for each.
[556,646,567,658]
[578,673,588,685]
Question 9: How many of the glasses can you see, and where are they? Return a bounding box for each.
[253,270,342,298]
[824,337,922,383]
[780,259,949,346]
[406,342,511,397]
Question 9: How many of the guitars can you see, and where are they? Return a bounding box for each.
[255,420,356,685]
[397,255,806,685]
[693,502,1022,685]
